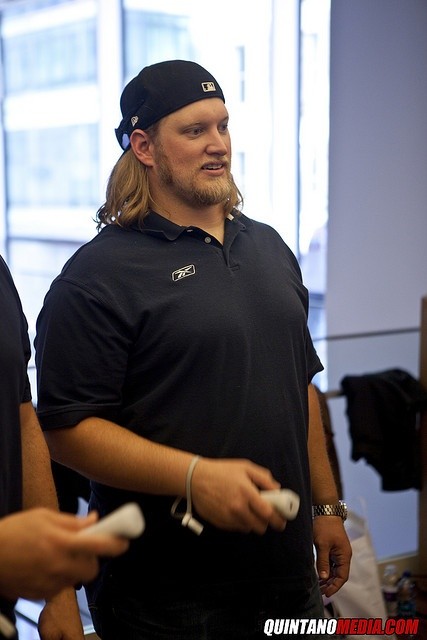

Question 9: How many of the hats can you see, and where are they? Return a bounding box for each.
[120,60,225,151]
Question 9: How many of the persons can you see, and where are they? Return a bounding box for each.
[0,252,129,640]
[34,60,352,639]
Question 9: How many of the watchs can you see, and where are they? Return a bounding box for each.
[312,499,348,520]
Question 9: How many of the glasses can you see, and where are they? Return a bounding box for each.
[115,99,146,151]
